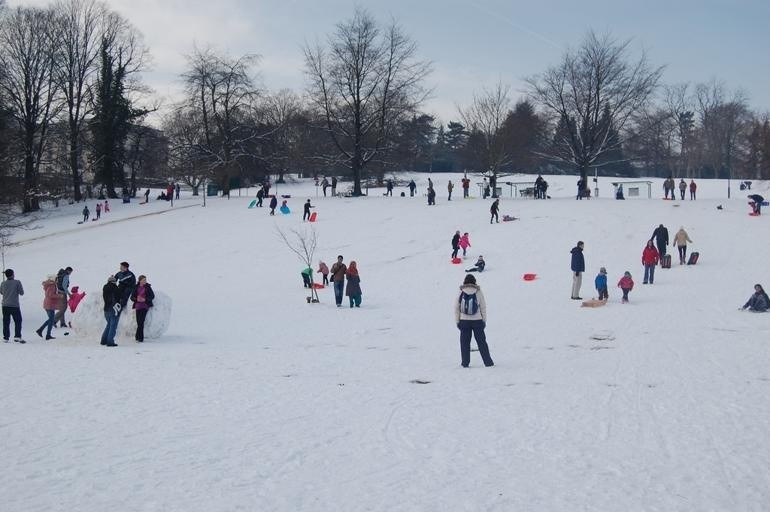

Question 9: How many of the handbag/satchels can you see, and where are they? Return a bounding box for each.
[329,265,343,282]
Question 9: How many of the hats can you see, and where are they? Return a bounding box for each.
[71,286,80,293]
[5,269,14,278]
[108,275,116,281]
[48,273,57,282]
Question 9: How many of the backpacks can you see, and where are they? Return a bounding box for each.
[458,291,479,315]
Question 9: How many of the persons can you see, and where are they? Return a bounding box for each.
[641,239,660,285]
[451,230,462,258]
[1,260,154,347]
[568,241,586,301]
[453,274,496,368]
[301,267,313,289]
[616,271,635,305]
[79,168,767,226]
[316,259,330,286]
[672,224,695,265]
[457,232,472,258]
[331,254,348,307]
[737,282,770,314]
[650,223,670,262]
[345,260,363,308]
[464,255,486,273]
[594,266,609,302]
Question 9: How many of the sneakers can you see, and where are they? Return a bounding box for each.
[3,335,27,344]
[101,338,118,347]
[37,329,56,342]
